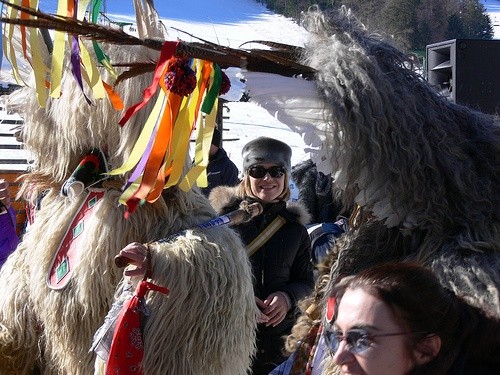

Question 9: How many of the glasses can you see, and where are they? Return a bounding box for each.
[247,166,287,178]
[322,329,429,353]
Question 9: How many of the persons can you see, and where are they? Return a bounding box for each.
[0,22,500,375]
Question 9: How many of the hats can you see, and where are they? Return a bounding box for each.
[241,135,292,173]
[212,128,221,147]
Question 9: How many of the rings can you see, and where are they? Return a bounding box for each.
[142,255,147,263]
